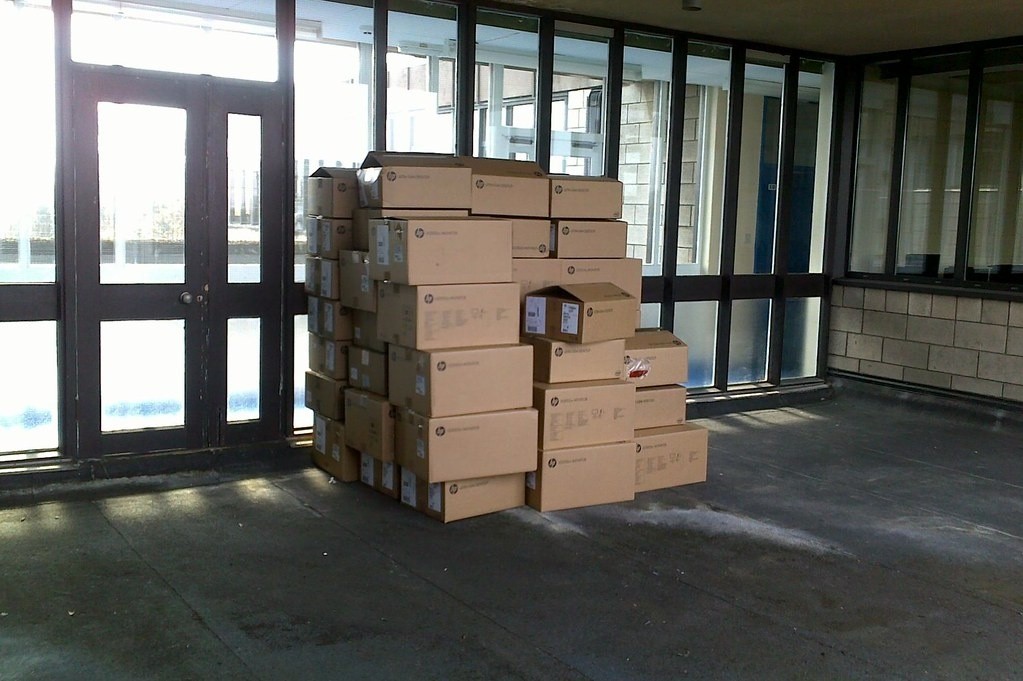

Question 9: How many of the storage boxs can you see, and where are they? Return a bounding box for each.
[304,150,709,525]
